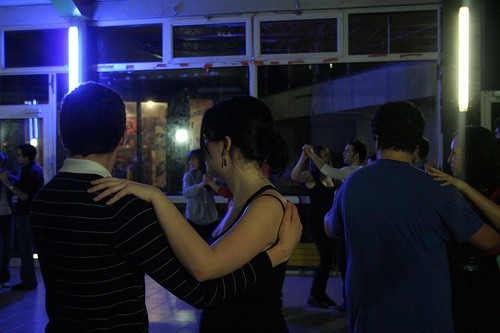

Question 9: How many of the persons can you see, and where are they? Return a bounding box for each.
[203,174,233,203]
[302,138,370,311]
[26,82,303,333]
[0,144,44,290]
[182,149,219,228]
[87,94,287,333]
[426,126,500,333]
[291,144,347,309]
[325,100,500,333]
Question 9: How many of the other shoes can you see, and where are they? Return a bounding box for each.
[307,297,329,308]
[12,282,38,290]
[2,282,11,287]
[336,305,347,311]
[322,294,336,306]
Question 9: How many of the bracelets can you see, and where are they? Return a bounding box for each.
[10,187,14,190]
